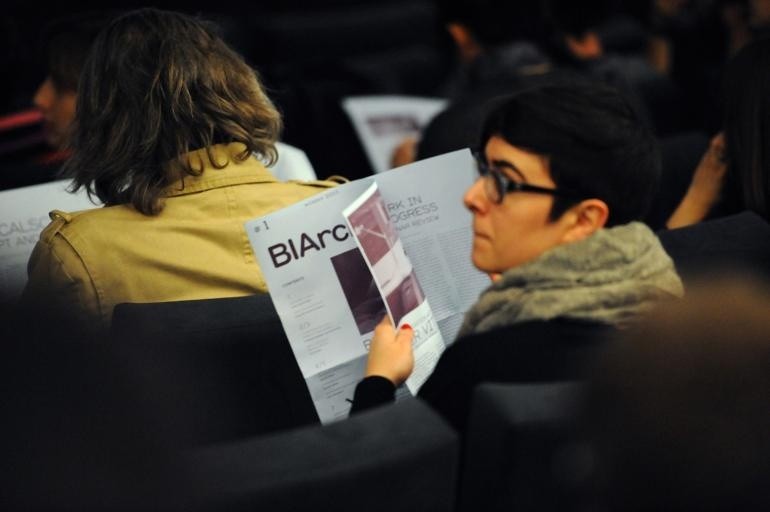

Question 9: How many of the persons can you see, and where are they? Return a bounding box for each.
[26,1,647,171]
[662,21,770,228]
[17,5,352,338]
[345,67,685,423]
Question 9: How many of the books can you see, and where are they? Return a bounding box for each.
[245,146,492,425]
[1,141,319,298]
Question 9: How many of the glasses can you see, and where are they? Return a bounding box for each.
[478,159,555,203]
